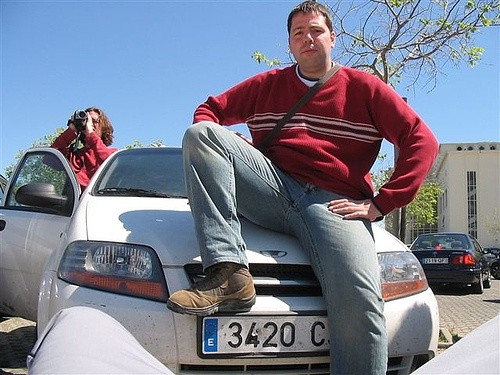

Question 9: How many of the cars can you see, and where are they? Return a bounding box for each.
[0,139,441,375]
[405,232,499,294]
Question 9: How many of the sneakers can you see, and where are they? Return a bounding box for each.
[167,263,257,317]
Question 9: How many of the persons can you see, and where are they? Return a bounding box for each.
[42,107,118,217]
[167,0,439,375]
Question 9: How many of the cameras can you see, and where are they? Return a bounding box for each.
[71,110,88,131]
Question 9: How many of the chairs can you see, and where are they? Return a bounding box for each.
[451,241,463,249]
[416,241,434,249]
